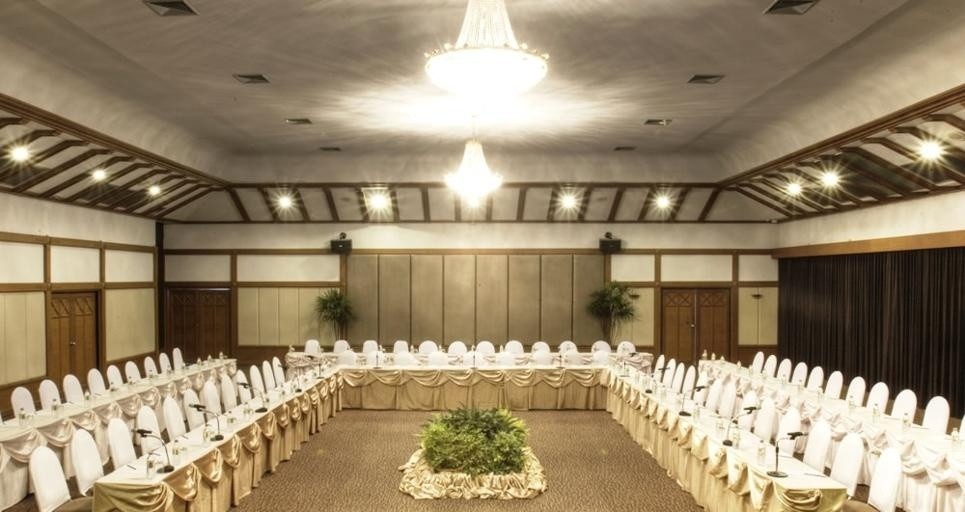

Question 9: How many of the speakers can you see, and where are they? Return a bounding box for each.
[600,238,620,252]
[330,239,352,253]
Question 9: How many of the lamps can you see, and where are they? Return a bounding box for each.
[425,0,549,93]
[444,132,503,210]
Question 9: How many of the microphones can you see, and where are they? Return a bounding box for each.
[131,428,151,433]
[630,352,639,356]
[278,364,287,370]
[744,406,761,410]
[471,345,477,353]
[558,352,564,359]
[189,403,205,408]
[376,353,380,360]
[788,431,809,436]
[305,354,314,358]
[658,367,670,370]
[696,385,708,389]
[237,382,249,386]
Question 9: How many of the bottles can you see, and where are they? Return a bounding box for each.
[619,350,960,460]
[145,367,324,471]
[288,343,627,371]
[20,351,225,430]
